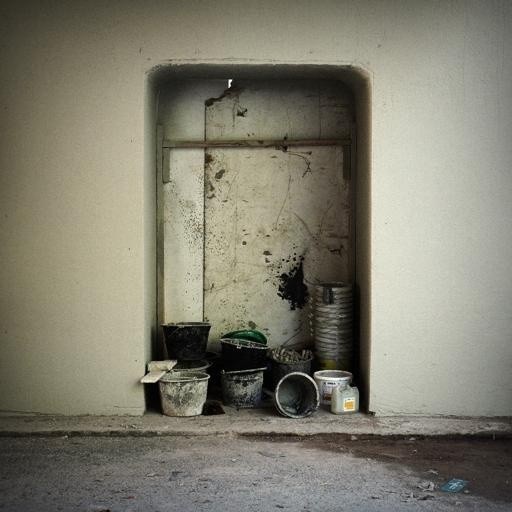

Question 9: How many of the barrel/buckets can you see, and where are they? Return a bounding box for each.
[166,359,210,371]
[274,371,320,419]
[272,354,314,379]
[222,366,268,409]
[157,370,211,418]
[219,337,270,372]
[159,321,212,361]
[308,281,354,371]
[328,384,360,415]
[313,369,353,406]
